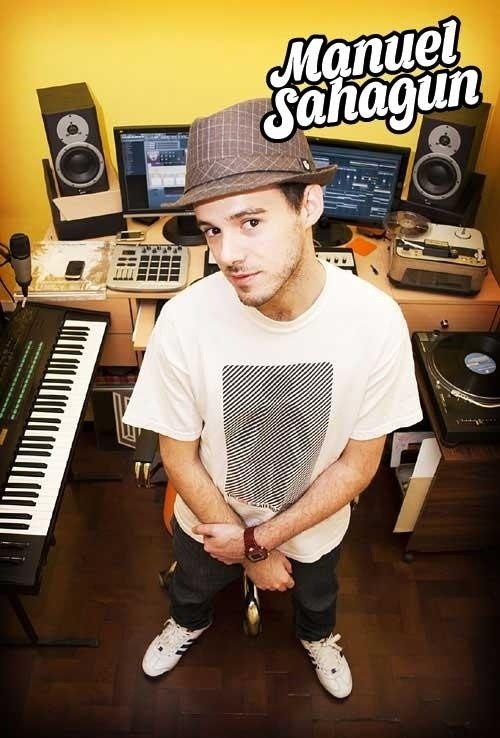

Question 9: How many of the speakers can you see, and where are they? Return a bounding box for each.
[407,102,491,207]
[37,83,110,197]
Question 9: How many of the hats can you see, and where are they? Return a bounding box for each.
[161,99,338,209]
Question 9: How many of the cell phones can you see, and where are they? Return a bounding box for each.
[115,230,145,240]
[65,260,83,280]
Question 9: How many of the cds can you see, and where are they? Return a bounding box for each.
[382,210,429,236]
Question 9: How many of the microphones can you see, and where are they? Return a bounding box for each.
[8,231,33,294]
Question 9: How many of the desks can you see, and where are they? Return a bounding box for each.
[12,193,500,435]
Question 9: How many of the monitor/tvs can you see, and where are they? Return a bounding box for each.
[304,135,411,246]
[113,125,209,246]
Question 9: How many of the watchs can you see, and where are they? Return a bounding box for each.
[243,525,270,563]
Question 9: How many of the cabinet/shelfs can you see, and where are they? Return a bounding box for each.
[387,330,500,563]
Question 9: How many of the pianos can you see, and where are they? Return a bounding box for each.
[0,300,120,650]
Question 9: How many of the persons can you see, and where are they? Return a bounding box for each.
[119,97,425,701]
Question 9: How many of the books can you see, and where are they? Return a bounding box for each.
[13,239,112,301]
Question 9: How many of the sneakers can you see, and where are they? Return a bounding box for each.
[300,632,353,699]
[142,616,212,676]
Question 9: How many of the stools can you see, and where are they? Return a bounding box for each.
[134,427,363,642]
